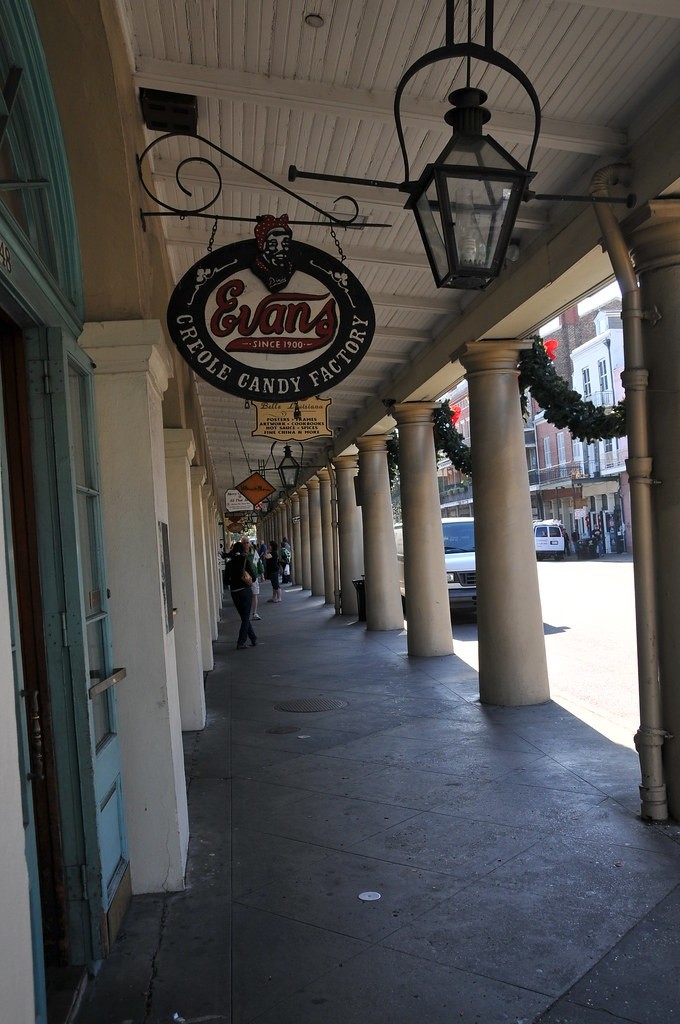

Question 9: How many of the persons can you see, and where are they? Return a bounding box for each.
[223,541,257,649]
[218,537,292,620]
[562,525,602,559]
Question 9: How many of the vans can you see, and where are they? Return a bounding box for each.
[395,517,478,624]
[534,524,565,561]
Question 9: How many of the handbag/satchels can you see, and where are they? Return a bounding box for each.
[283,563,290,575]
[242,571,259,587]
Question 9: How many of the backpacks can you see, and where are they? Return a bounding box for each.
[277,544,292,565]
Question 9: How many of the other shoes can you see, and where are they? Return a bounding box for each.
[274,599,282,602]
[253,613,261,620]
[252,638,258,645]
[267,599,274,602]
[237,644,250,649]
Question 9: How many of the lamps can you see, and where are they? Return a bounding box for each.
[394,0,541,292]
[271,439,304,490]
[243,497,270,532]
